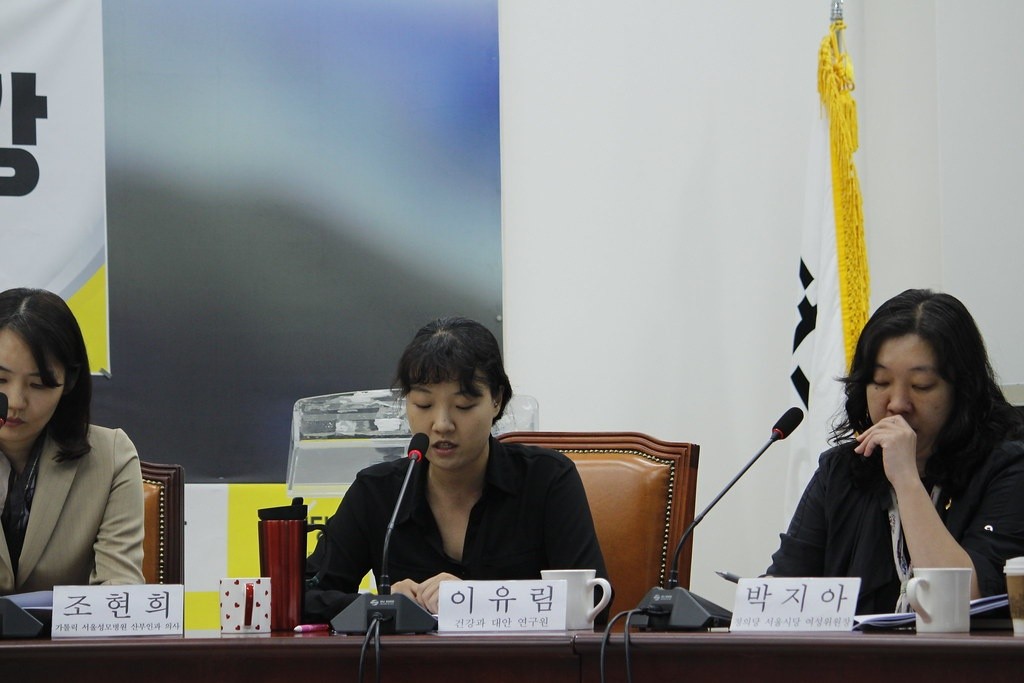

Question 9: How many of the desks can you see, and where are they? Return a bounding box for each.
[573,629,1024,682]
[2,628,578,683]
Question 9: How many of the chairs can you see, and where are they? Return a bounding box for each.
[493,429,701,633]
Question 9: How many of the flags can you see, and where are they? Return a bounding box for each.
[778,22,879,465]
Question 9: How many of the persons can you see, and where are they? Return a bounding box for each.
[306,318,615,626]
[0,286,146,593]
[757,287,1024,615]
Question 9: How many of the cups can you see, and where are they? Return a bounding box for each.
[256,496,335,631]
[1002,556,1024,636]
[540,569,611,633]
[905,568,973,634]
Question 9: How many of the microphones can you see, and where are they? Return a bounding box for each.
[628,406,804,631]
[330,432,438,635]
[0,393,45,639]
